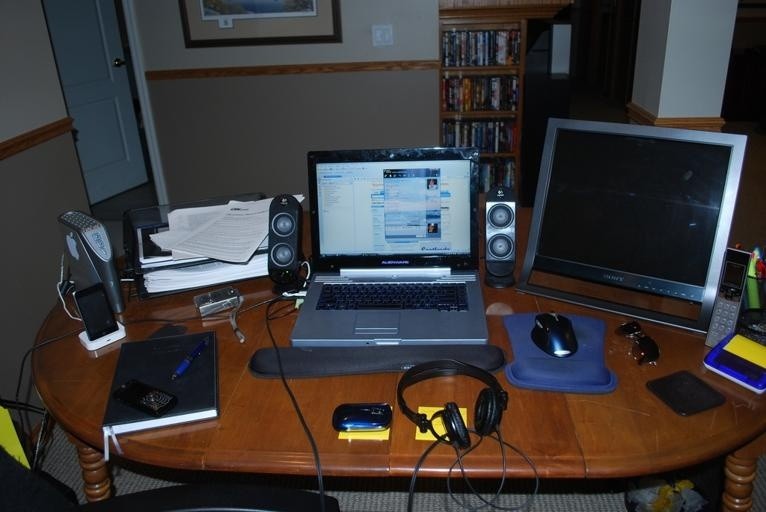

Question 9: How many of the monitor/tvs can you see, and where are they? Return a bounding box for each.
[515,117,747,334]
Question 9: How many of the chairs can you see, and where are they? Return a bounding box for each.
[0,396,341,512]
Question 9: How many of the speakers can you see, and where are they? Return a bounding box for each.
[484,185,515,287]
[268,194,303,293]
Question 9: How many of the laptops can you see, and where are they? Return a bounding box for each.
[288,145,489,347]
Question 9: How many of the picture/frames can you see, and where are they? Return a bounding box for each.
[177,0,343,49]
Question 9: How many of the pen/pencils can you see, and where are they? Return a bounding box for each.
[170,335,210,382]
[735,242,766,308]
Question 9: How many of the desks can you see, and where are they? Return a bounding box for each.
[32,210,766,511]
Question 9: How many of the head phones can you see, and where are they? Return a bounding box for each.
[397,358,508,449]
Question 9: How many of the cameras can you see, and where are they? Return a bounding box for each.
[192,285,240,318]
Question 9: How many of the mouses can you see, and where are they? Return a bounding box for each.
[531,311,578,358]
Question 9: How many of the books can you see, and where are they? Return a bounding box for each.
[100,330,220,437]
[441,26,521,192]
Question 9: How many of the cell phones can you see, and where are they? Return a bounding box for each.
[74,283,119,342]
[332,403,392,431]
[705,248,752,348]
[111,378,178,418]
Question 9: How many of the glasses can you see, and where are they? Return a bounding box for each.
[614,320,661,366]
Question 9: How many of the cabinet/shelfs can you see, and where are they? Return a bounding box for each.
[439,1,571,209]
